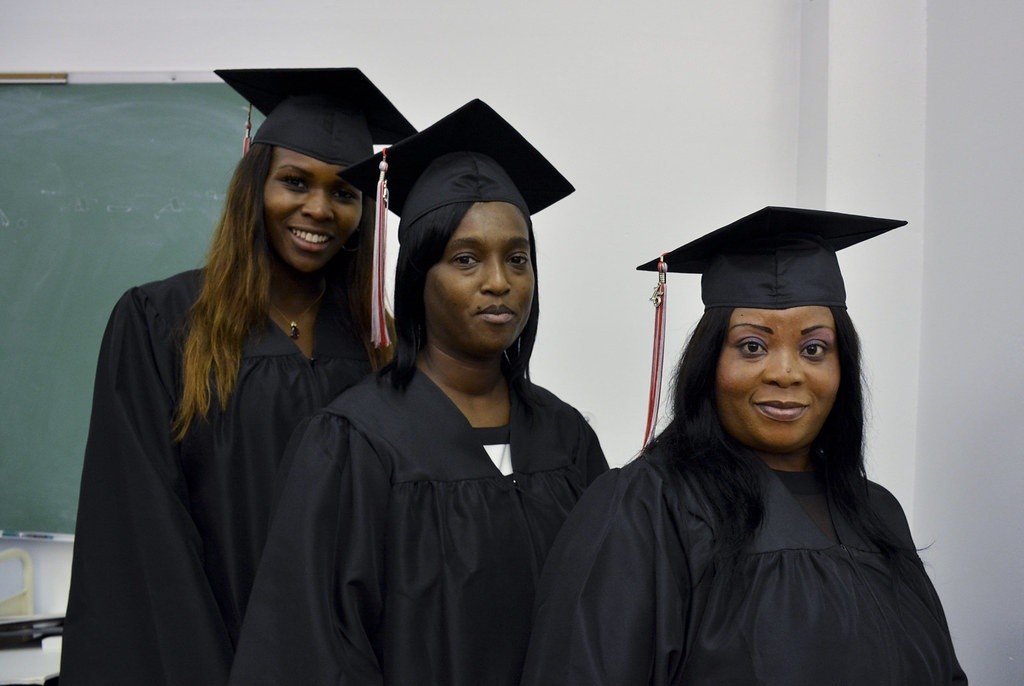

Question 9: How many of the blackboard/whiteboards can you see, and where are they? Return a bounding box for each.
[0,74,267,541]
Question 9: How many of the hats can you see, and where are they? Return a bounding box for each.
[636,206,909,453]
[213,67,417,165]
[335,99,576,350]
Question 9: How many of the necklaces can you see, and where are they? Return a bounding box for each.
[271,281,326,339]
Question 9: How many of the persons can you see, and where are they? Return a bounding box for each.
[58,67,420,686]
[231,98,612,686]
[518,206,968,686]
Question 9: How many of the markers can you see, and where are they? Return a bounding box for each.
[0,530,53,539]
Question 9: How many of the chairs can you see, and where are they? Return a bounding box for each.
[0,548,33,617]
